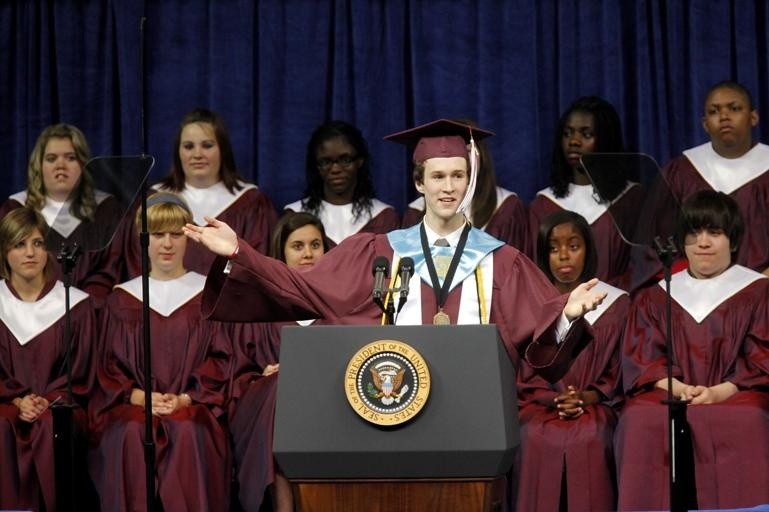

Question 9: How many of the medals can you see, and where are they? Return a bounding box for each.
[431,304,451,324]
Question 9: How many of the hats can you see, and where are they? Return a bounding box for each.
[383,118,495,214]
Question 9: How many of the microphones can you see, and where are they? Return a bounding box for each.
[370,255,390,300]
[398,257,414,302]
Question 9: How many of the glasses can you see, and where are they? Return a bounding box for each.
[317,154,361,170]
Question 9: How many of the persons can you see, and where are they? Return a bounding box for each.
[0,206,93,511]
[179,116,607,369]
[0,126,126,321]
[669,78,769,277]
[87,190,231,511]
[125,104,276,275]
[400,121,526,252]
[525,95,670,291]
[274,121,400,252]
[513,210,635,511]
[614,190,769,510]
[228,213,335,510]
